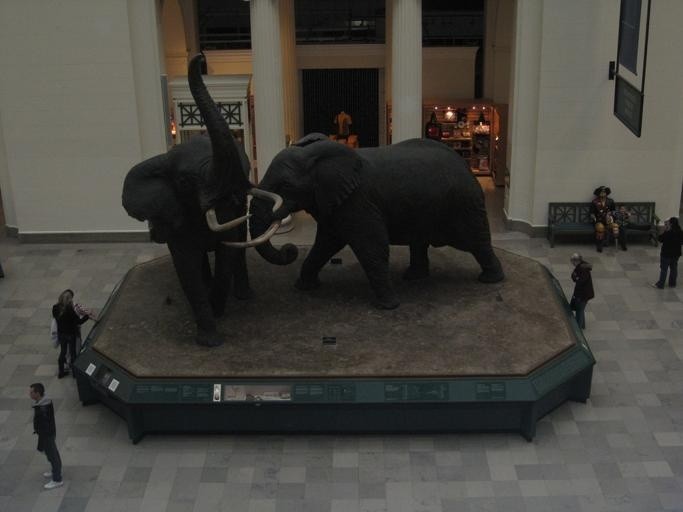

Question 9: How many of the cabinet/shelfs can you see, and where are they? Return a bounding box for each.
[425,101,507,187]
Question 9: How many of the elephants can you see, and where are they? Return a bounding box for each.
[220,132,505,310]
[121,54,284,348]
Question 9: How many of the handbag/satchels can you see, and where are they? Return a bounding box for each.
[51,317,58,349]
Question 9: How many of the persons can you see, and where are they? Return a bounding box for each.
[52,289,95,379]
[570,253,594,329]
[652,217,683,289]
[30,383,64,489]
[589,186,631,252]
[334,112,352,137]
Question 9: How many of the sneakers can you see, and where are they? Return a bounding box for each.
[58,372,69,378]
[43,471,63,489]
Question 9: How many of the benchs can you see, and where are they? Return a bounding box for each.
[549,202,660,247]
